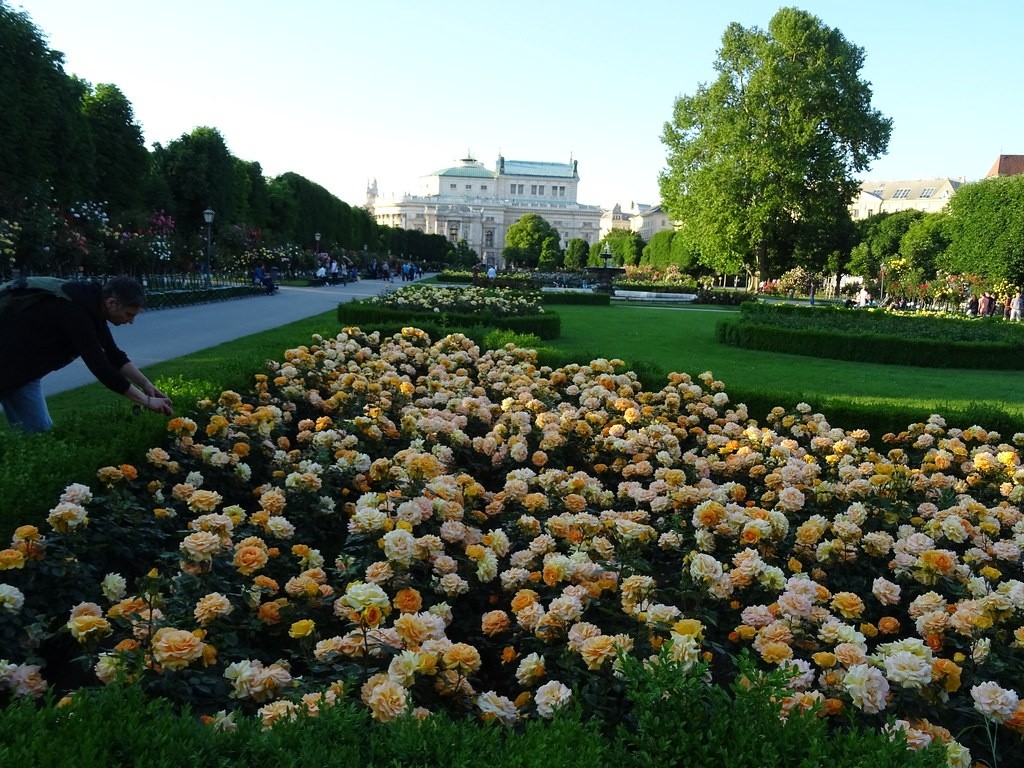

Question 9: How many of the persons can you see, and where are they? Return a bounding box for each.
[252,262,279,295]
[472,264,480,286]
[967,291,1023,321]
[397,259,441,281]
[367,258,395,283]
[316,257,358,286]
[553,272,561,287]
[856,285,906,311]
[810,282,815,305]
[0,277,173,435]
[487,264,495,288]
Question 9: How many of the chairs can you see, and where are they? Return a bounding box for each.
[67,270,280,314]
[307,270,351,287]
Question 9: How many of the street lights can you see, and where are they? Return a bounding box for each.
[314,232,322,259]
[745,262,750,292]
[880,261,887,301]
[204,208,215,285]
